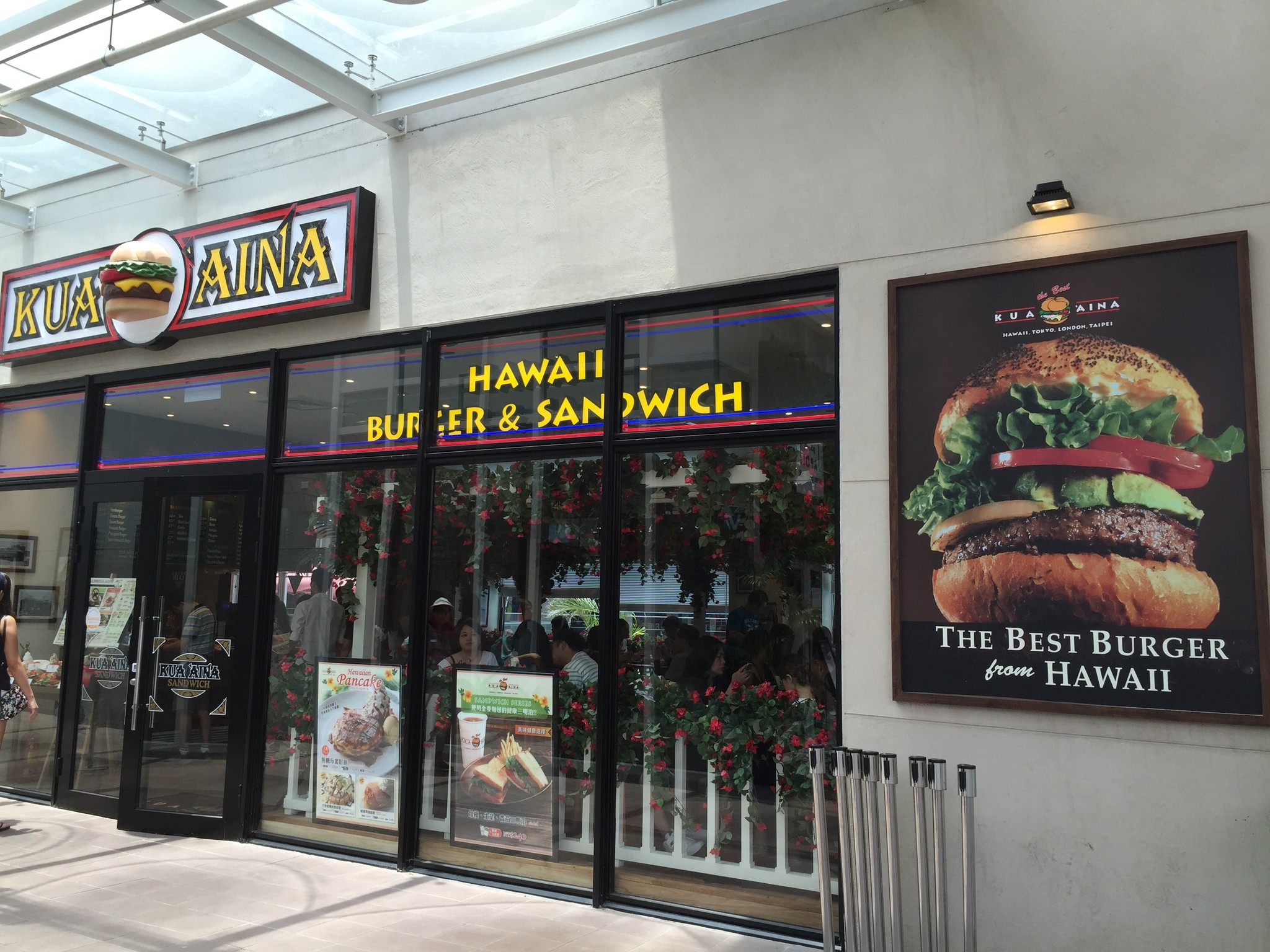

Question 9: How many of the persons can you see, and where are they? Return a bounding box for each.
[285,568,346,671]
[382,598,636,698]
[0,572,39,831]
[652,590,835,708]
[160,592,217,759]
[271,587,355,657]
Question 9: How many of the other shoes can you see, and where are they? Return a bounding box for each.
[0,822,10,831]
[178,752,192,765]
[195,752,213,763]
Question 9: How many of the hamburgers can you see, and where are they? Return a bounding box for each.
[901,338,1244,629]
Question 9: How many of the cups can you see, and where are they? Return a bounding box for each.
[511,658,519,667]
[457,711,488,768]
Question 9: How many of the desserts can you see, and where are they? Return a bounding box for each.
[363,777,395,810]
[325,773,354,805]
[330,682,399,756]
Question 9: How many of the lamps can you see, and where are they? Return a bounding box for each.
[1026,181,1075,215]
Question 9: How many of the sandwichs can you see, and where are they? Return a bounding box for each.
[498,749,549,795]
[470,757,511,804]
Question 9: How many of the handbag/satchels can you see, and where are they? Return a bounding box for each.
[0,686,28,720]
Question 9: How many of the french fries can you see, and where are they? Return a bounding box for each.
[497,732,522,763]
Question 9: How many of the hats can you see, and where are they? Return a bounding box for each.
[430,597,454,611]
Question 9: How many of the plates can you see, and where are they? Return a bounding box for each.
[318,690,399,777]
[460,749,552,805]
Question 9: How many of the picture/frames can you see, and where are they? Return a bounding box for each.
[0,534,38,573]
[13,585,60,624]
